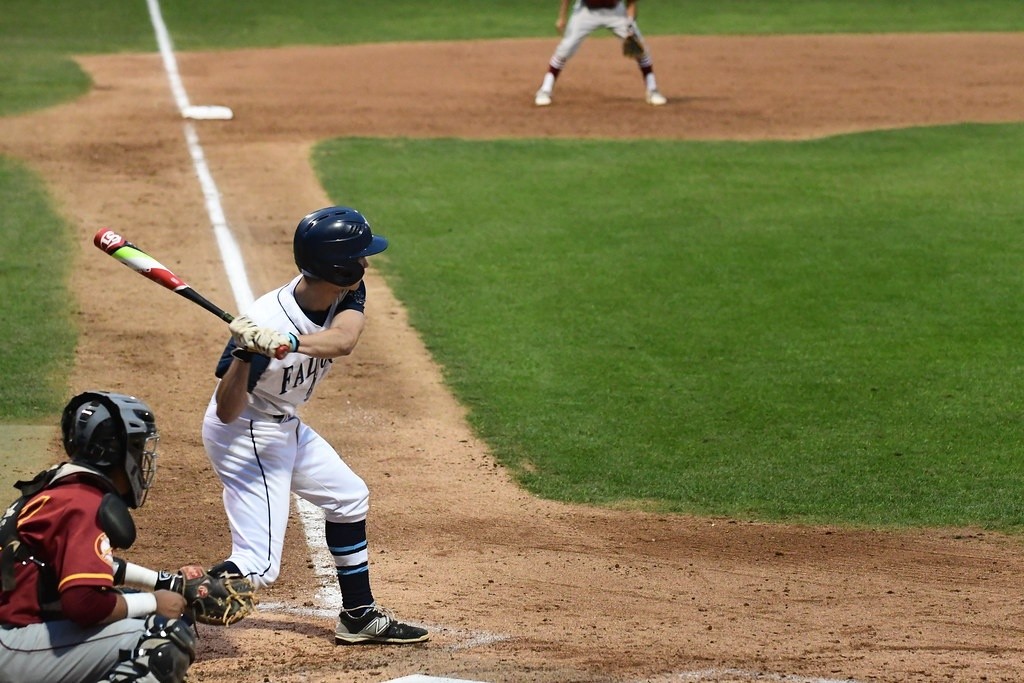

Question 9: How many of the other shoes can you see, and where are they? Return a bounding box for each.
[644,92,667,105]
[535,89,552,106]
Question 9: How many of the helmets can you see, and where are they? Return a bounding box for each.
[293,206,388,287]
[61,391,160,509]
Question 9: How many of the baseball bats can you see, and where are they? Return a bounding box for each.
[93,226,289,360]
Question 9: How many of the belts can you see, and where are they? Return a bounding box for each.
[273,415,284,423]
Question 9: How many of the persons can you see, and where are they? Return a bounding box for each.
[534,0,668,107]
[200,205,430,646]
[0,390,258,683]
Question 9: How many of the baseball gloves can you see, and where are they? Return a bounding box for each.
[177,565,260,628]
[622,31,645,61]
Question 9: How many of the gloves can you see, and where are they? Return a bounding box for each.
[229,316,260,362]
[254,328,300,358]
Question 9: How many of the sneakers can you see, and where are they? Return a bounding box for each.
[334,604,429,645]
